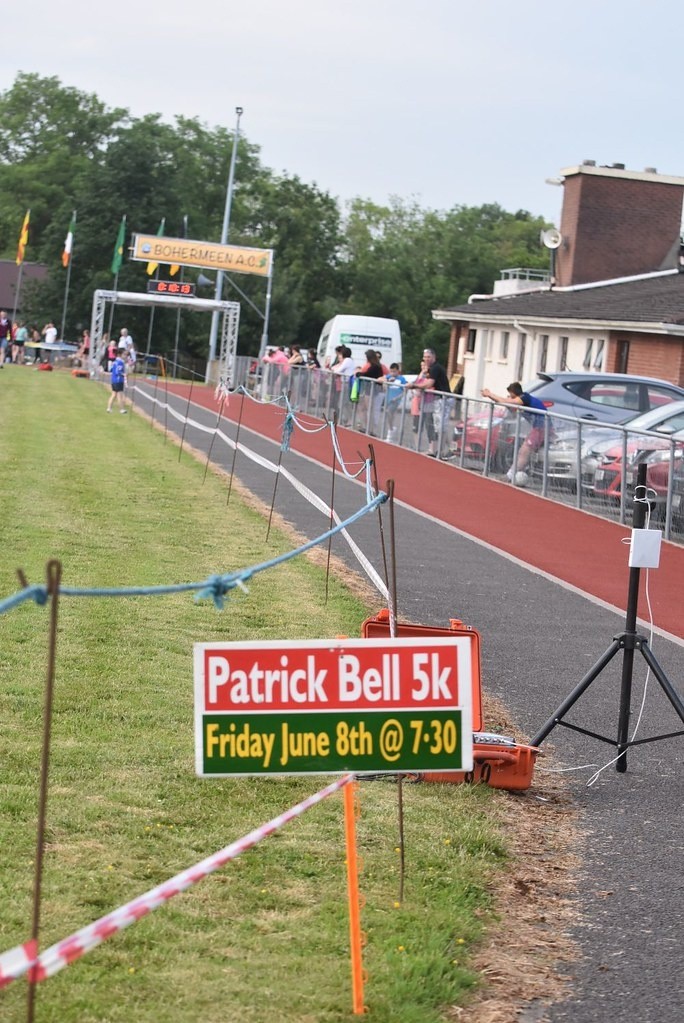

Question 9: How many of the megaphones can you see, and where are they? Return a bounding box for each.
[542,229,565,249]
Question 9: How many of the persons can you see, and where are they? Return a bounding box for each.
[250,344,553,481]
[0,311,136,414]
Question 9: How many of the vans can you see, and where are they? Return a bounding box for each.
[314,314,403,374]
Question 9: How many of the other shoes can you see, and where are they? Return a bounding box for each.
[368,431,377,437]
[441,449,456,460]
[495,474,511,483]
[0,365,4,368]
[426,450,438,458]
[106,409,112,412]
[386,426,397,441]
[120,409,127,414]
[21,360,26,364]
[12,360,18,363]
[357,428,366,433]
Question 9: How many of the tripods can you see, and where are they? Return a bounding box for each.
[529,462,684,771]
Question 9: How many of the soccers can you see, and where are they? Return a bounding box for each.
[515,471,528,487]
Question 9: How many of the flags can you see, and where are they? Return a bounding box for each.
[62,215,77,268]
[111,219,126,274]
[169,215,187,276]
[15,212,30,266]
[147,220,164,275]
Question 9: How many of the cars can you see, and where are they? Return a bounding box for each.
[259,345,309,386]
[394,374,418,413]
[452,370,684,538]
